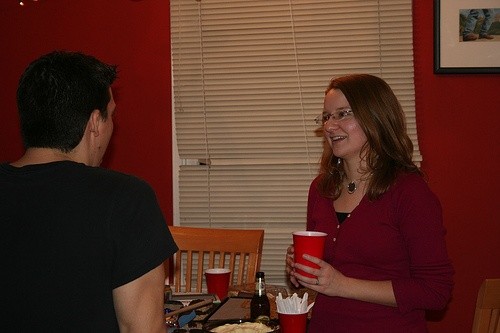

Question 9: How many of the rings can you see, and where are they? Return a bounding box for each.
[315,278,319,285]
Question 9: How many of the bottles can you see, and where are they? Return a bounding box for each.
[250,272,270,322]
[165,285,172,300]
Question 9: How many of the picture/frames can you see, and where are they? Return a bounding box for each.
[433,0,500,75]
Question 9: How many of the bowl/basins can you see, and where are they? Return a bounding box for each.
[204,320,280,333]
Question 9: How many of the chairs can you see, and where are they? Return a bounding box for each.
[168,225,264,294]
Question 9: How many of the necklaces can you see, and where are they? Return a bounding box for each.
[345,171,366,193]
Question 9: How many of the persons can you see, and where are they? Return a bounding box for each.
[286,74,453,333]
[0,53,179,333]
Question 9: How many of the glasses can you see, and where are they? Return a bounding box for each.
[313,108,353,126]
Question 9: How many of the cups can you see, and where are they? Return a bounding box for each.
[276,309,309,333]
[291,230,328,284]
[205,268,232,302]
